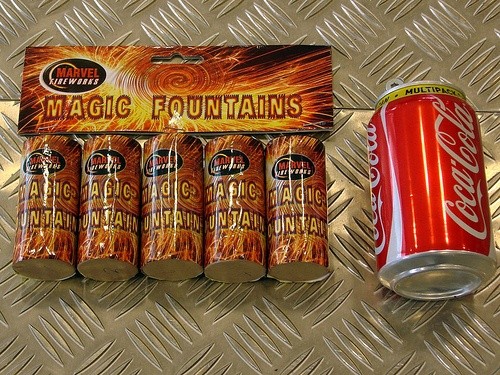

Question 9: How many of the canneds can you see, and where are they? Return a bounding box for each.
[366,80,499,303]
